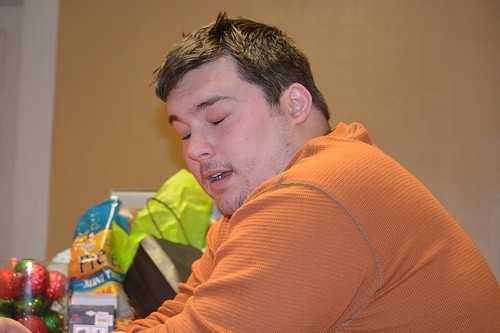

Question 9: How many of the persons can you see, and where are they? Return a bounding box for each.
[110,9,500,333]
